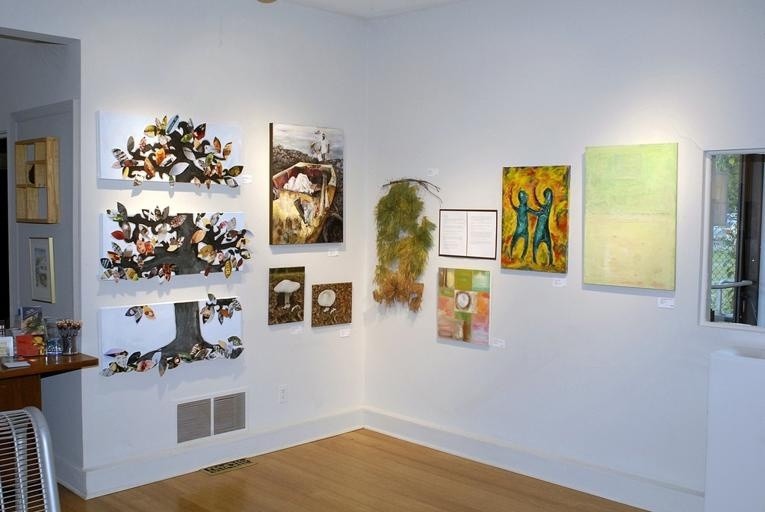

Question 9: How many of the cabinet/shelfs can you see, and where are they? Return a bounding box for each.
[14,136,59,225]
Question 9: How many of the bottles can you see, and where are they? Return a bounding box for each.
[46,322,64,355]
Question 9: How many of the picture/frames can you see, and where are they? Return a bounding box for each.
[438,208,498,260]
[28,235,56,304]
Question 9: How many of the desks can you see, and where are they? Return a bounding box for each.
[0,352,98,511]
